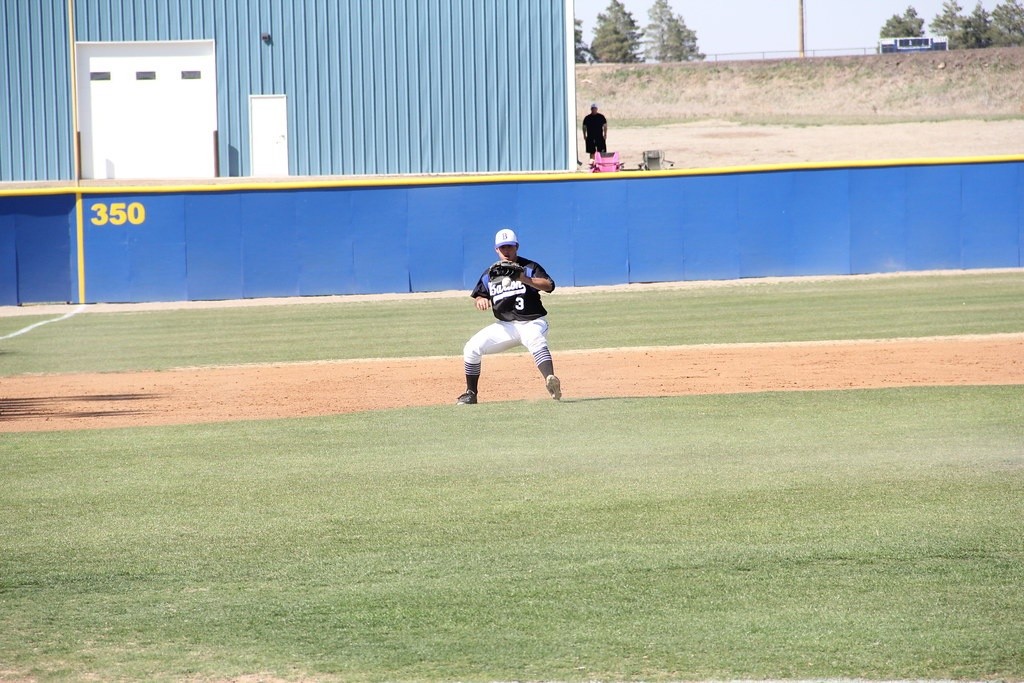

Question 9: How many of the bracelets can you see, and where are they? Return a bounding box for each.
[605,135,606,136]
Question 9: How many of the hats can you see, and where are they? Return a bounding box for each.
[494,229,519,249]
[590,103,599,109]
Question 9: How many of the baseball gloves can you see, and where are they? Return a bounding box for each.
[488,260,525,283]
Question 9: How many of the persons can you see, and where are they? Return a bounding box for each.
[457,229,562,405]
[582,104,607,160]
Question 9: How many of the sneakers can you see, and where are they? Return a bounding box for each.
[545,374,562,401]
[457,389,478,405]
